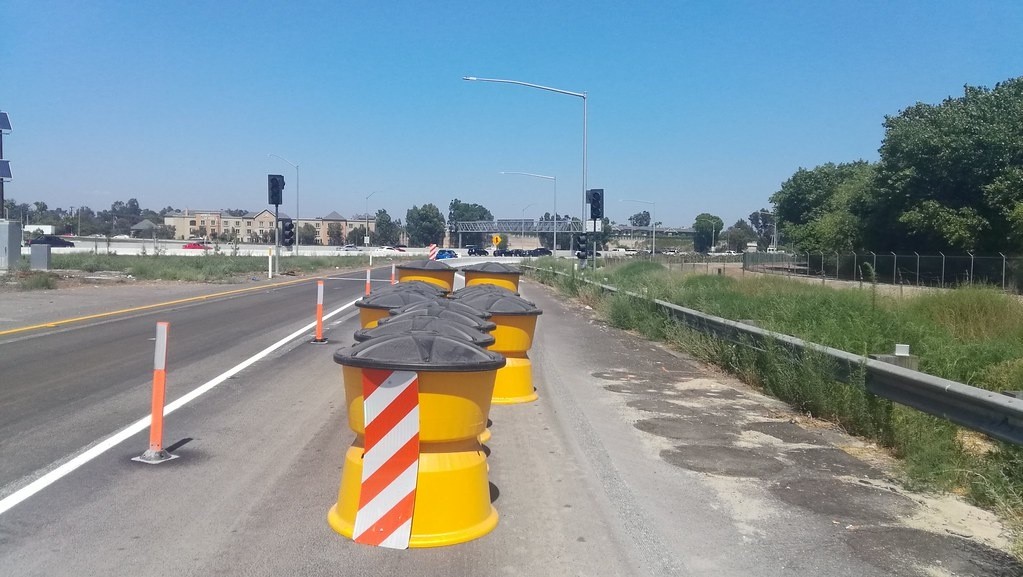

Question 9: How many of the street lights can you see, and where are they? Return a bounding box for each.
[699,217,730,251]
[267,153,304,256]
[497,172,557,261]
[618,199,656,256]
[366,190,377,248]
[766,223,777,252]
[462,76,589,231]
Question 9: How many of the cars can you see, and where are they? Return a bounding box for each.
[722,250,740,255]
[436,248,458,260]
[493,248,552,257]
[334,244,364,252]
[378,244,408,253]
[180,241,213,250]
[662,249,679,256]
[468,247,489,257]
[27,234,76,248]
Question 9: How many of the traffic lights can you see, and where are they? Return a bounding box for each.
[590,189,605,219]
[576,233,588,260]
[282,218,294,247]
[268,174,284,204]
